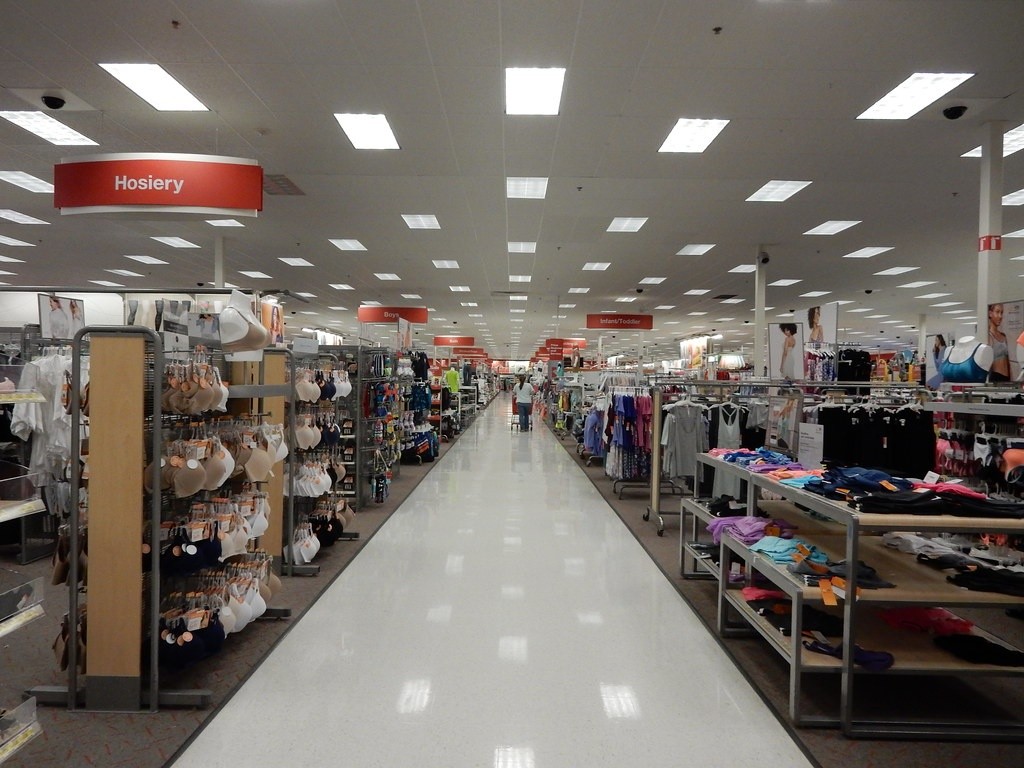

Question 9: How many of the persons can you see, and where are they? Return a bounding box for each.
[925,334,947,390]
[779,323,797,380]
[505,377,510,393]
[512,376,536,433]
[217,288,271,362]
[808,306,824,343]
[47,295,87,342]
[443,367,461,395]
[268,305,284,346]
[987,303,1011,404]
[195,312,219,339]
[940,336,994,383]
[463,361,472,386]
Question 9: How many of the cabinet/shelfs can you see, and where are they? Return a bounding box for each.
[679,448,1024,744]
[0,287,502,768]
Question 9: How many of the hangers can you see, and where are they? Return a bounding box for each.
[840,340,863,366]
[937,418,971,438]
[655,367,753,387]
[604,367,636,380]
[973,420,1024,448]
[803,340,836,359]
[849,395,883,415]
[895,395,936,415]
[802,395,848,414]
[709,394,751,415]
[661,392,709,412]
[592,387,651,411]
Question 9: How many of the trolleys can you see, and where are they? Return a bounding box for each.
[509,394,534,432]
